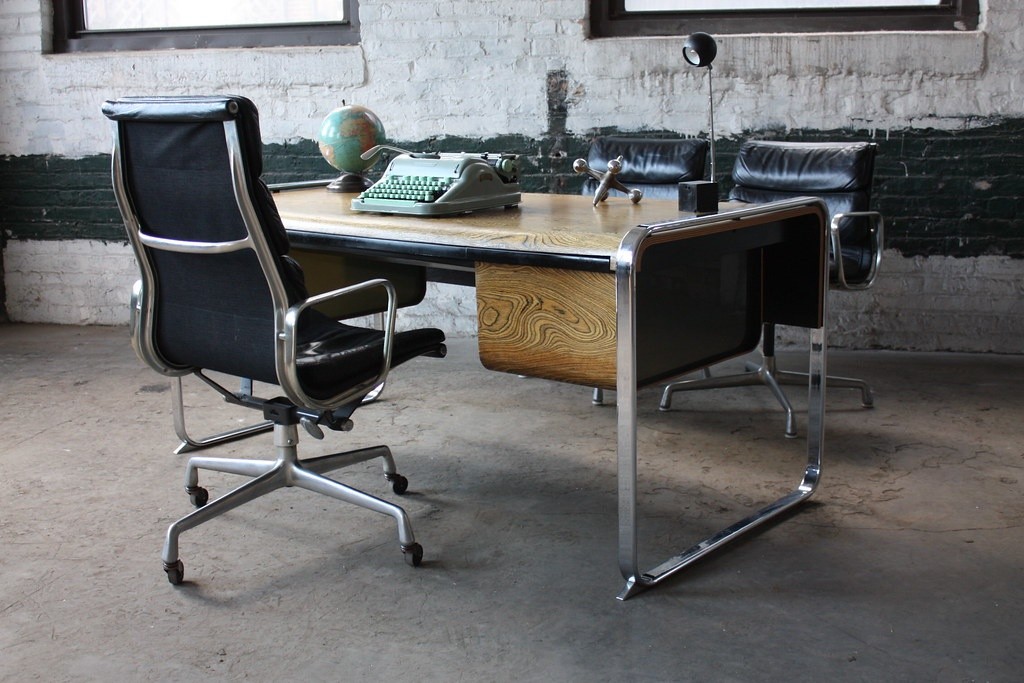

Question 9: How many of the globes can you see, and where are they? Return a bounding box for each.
[315,98,386,195]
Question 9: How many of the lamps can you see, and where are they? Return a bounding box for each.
[681,31,716,183]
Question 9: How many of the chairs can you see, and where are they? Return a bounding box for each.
[660,139,884,438]
[520,137,711,408]
[100,94,447,584]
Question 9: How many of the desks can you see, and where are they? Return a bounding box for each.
[167,186,832,599]
